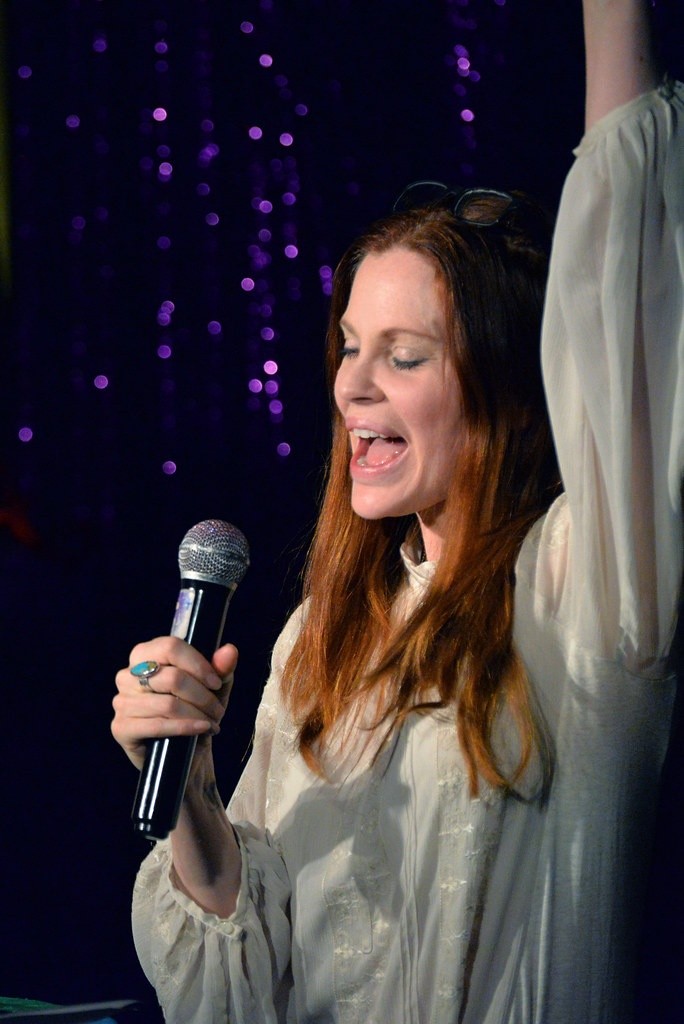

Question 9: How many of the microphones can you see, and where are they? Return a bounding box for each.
[133,520,251,841]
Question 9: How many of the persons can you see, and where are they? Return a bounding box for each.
[107,1,684,1024]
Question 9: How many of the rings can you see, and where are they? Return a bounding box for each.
[131,660,160,694]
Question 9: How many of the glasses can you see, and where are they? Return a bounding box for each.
[394,182,519,235]
